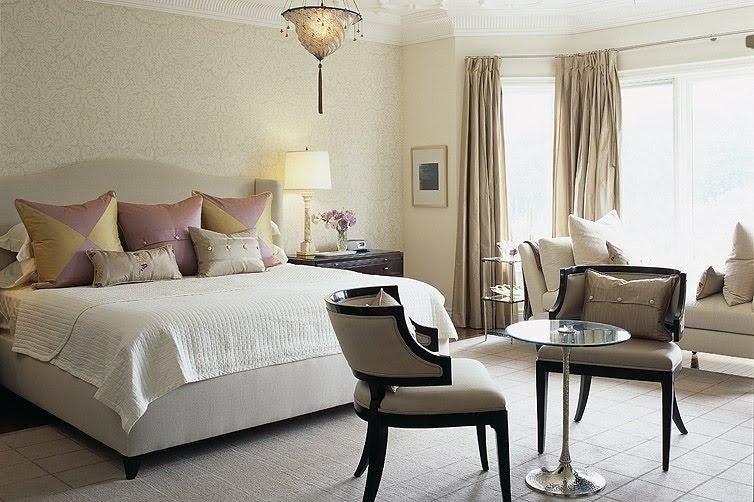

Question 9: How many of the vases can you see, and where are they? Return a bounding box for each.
[336,229,348,252]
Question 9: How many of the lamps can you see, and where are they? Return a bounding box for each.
[279,0,364,117]
[282,146,333,255]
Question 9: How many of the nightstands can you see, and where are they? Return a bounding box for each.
[286,248,404,278]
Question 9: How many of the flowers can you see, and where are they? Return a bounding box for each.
[311,207,357,238]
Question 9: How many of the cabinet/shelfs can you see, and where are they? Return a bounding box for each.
[480,254,527,343]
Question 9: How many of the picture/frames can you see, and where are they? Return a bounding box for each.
[411,144,450,209]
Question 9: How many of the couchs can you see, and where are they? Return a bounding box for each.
[516,237,753,361]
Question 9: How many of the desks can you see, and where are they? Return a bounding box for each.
[504,319,632,498]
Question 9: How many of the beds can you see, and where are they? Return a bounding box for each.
[1,160,460,480]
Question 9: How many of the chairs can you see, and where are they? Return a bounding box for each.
[324,284,511,502]
[535,264,688,471]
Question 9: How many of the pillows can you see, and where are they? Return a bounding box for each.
[537,236,575,291]
[188,225,265,276]
[0,256,37,290]
[14,189,126,288]
[568,207,628,267]
[85,243,183,288]
[116,194,204,276]
[605,239,657,264]
[694,265,725,300]
[191,189,273,265]
[580,268,680,342]
[366,287,413,393]
[0,220,36,261]
[722,222,754,306]
